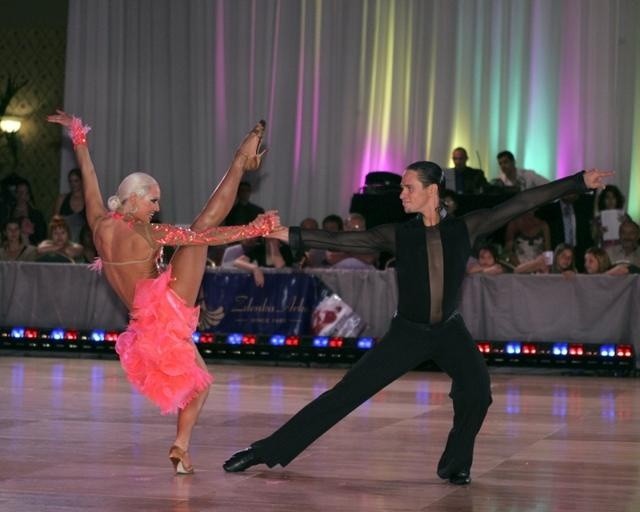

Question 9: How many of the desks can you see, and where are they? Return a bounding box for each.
[2,262,639,350]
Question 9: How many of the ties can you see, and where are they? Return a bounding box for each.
[564,205,573,248]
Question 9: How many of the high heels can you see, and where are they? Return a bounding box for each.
[168,446,194,476]
[239,119,267,173]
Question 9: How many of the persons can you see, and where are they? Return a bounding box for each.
[222,160,615,484]
[485,149,554,189]
[1,167,639,291]
[46,108,284,474]
[441,147,505,197]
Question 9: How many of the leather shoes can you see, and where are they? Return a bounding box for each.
[222,447,264,472]
[437,457,471,485]
